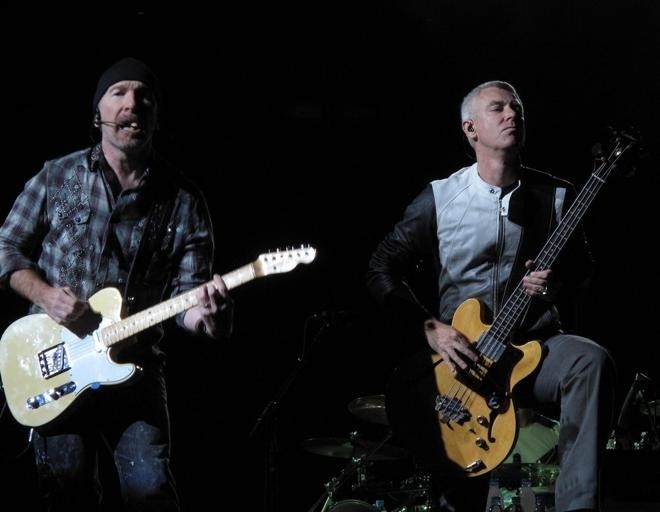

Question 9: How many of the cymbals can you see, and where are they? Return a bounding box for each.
[300,437,403,460]
[348,395,387,424]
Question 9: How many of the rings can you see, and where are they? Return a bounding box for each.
[541,286,548,295]
[203,302,209,309]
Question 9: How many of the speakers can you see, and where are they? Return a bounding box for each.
[599,451,660,512]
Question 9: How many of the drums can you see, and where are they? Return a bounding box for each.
[325,499,378,512]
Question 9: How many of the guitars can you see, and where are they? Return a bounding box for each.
[432,135,633,478]
[0,245,317,427]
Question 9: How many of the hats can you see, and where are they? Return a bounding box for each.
[92,56,165,115]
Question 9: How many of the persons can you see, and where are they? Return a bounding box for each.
[0,56,234,512]
[366,80,618,510]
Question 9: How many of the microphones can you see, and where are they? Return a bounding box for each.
[92,121,138,130]
[311,307,348,324]
